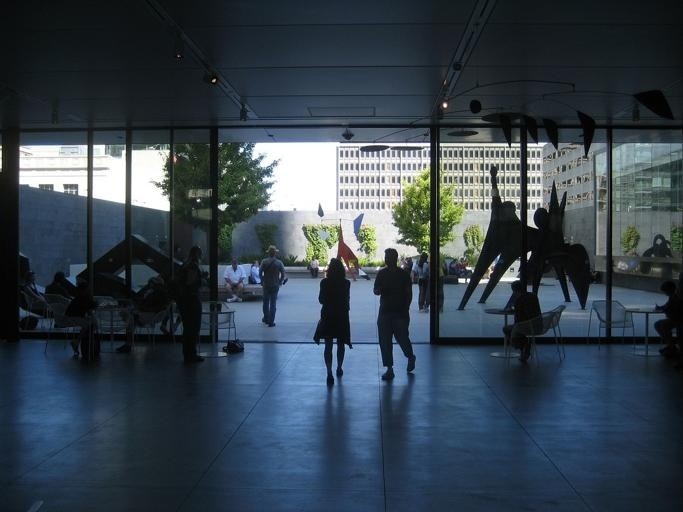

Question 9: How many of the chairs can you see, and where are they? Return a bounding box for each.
[508,311,561,369]
[587,299,636,354]
[21,283,237,365]
[529,304,566,358]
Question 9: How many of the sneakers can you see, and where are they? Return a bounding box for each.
[227,299,232,303]
[261,317,270,323]
[407,355,415,372]
[381,372,394,380]
[237,298,242,302]
[269,323,275,327]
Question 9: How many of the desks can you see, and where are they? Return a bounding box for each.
[484,308,520,358]
[625,307,662,356]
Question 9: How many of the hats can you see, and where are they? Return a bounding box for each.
[265,245,280,253]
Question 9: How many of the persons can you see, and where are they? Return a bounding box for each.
[313,258,354,385]
[400,257,419,284]
[310,256,318,278]
[654,280,683,354]
[18,271,167,354]
[503,280,541,363]
[173,245,204,366]
[224,260,261,302]
[372,248,416,381]
[258,246,285,327]
[449,258,469,278]
[417,252,431,311]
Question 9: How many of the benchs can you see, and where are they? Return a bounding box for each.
[199,284,264,301]
[286,269,327,279]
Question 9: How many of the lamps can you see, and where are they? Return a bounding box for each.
[438,54,464,117]
[174,37,250,121]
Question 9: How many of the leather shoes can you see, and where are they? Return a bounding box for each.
[184,356,203,362]
[336,370,342,376]
[327,378,333,385]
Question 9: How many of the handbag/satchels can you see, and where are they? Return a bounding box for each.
[222,339,244,353]
